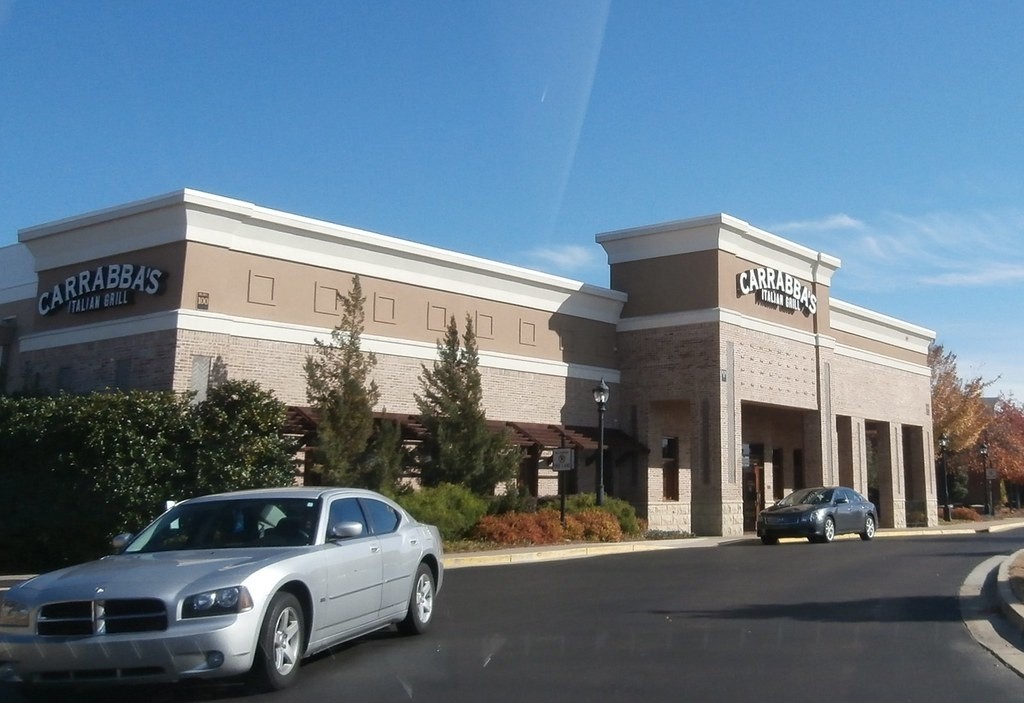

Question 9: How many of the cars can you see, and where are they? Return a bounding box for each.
[756,485,878,545]
[0,486,447,703]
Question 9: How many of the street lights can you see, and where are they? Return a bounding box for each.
[979,442,992,517]
[592,377,610,507]
[938,432,954,522]
